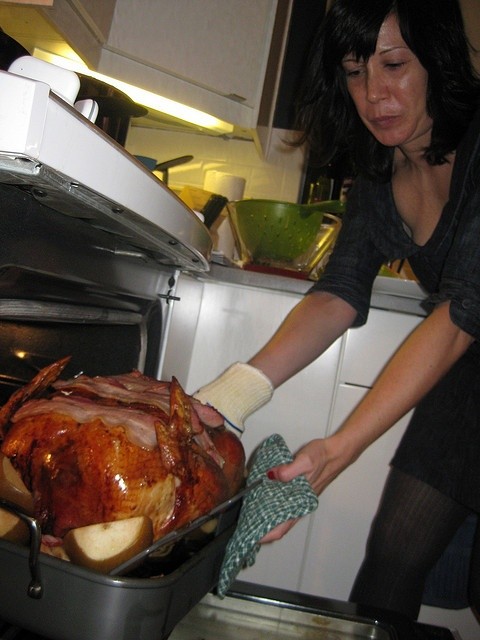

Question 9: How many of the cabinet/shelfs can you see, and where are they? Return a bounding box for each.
[296,276,428,611]
[43,1,119,73]
[98,1,293,165]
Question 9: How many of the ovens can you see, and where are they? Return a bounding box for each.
[1,75,261,635]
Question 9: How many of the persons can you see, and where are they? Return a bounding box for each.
[169,0,480,619]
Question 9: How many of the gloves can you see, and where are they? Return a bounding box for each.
[190,359,277,442]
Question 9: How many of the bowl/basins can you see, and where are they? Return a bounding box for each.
[227,195,343,279]
[290,212,341,277]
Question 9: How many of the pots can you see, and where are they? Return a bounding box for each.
[67,70,146,146]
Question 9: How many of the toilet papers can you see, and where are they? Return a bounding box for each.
[205,169,245,268]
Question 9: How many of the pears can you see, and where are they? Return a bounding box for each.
[61,514,155,577]
[1,453,33,511]
[1,510,31,546]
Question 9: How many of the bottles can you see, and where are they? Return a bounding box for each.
[199,158,246,203]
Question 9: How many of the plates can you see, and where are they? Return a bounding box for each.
[371,270,426,299]
